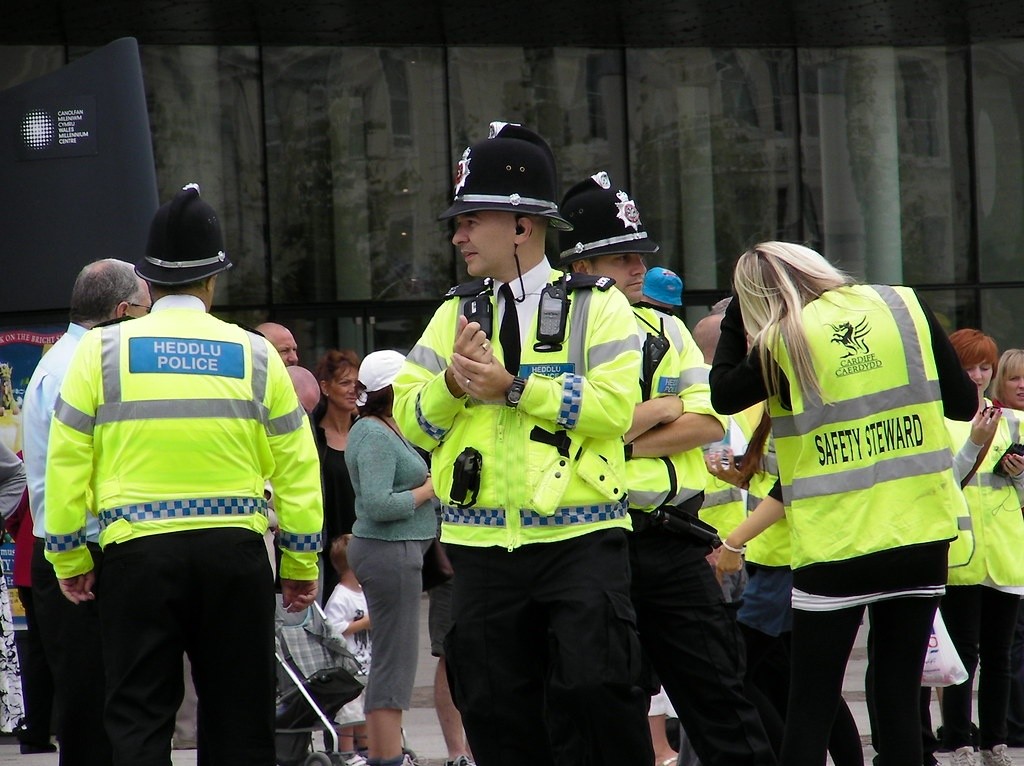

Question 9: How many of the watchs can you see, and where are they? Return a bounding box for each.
[504,376,525,406]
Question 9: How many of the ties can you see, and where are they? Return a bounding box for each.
[498,284,522,374]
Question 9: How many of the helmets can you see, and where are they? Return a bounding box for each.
[134,183,233,286]
[555,172,659,265]
[438,124,575,233]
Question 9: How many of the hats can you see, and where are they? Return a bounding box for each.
[642,269,683,305]
[354,348,405,407]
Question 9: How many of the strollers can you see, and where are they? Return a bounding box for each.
[273,592,418,766]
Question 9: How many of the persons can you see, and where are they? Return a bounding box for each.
[44,182,323,766]
[0,257,473,765]
[552,173,771,766]
[708,241,979,765]
[389,122,661,765]
[639,265,1024,766]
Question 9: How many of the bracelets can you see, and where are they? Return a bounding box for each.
[624,441,634,460]
[723,539,744,552]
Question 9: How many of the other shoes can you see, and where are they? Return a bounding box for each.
[20,744,58,754]
[368,751,417,766]
[978,743,1013,766]
[445,755,472,766]
[949,746,980,766]
[346,756,368,766]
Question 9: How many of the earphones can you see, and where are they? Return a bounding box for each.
[515,225,524,235]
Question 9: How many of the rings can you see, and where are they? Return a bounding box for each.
[466,379,471,387]
[481,342,490,351]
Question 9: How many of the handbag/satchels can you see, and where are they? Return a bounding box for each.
[274,663,364,730]
[921,607,968,687]
[422,536,454,592]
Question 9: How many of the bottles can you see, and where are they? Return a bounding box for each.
[702,418,731,470]
